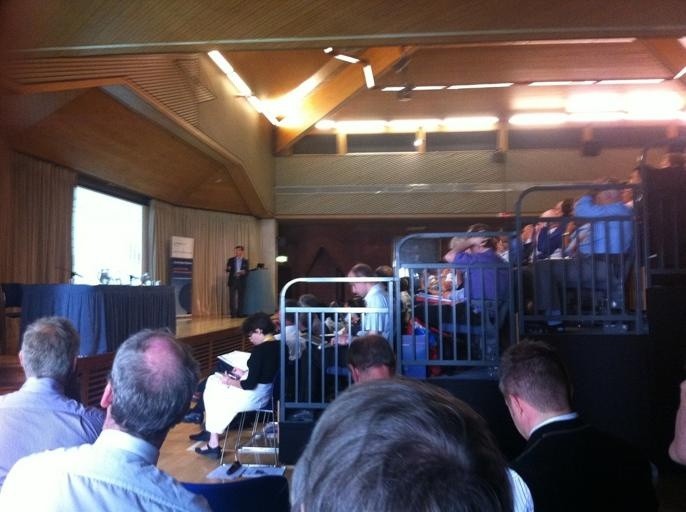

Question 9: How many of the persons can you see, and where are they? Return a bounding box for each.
[1,326,212,512]
[498,339,659,512]
[1,315,106,483]
[294,377,514,511]
[667,380,686,512]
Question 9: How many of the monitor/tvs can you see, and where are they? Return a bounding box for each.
[279,421,316,466]
[257,264,264,269]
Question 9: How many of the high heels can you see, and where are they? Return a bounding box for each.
[194,444,220,460]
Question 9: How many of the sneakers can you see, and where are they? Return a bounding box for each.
[182,408,203,423]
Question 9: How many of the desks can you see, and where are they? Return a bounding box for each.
[18,283,177,358]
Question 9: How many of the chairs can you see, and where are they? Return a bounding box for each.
[218,372,281,468]
[0,280,25,352]
[177,473,292,511]
[437,267,518,370]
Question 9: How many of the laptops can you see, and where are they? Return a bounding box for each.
[301,332,334,350]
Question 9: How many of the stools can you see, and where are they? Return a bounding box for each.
[326,364,354,402]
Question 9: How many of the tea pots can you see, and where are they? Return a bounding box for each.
[98,268,159,286]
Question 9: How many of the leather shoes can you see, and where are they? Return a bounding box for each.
[188,430,211,442]
